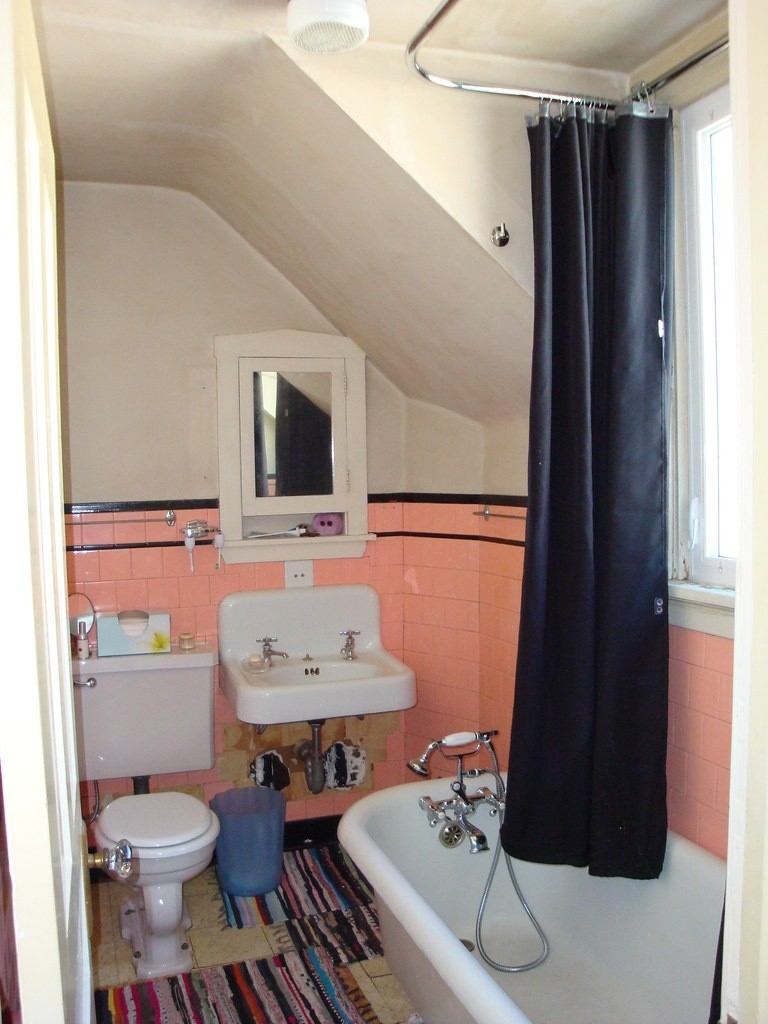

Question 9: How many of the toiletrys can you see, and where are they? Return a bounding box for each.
[246,525,310,539]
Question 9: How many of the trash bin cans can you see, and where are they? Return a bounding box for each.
[209,786,286,897]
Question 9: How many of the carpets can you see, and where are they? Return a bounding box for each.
[93,842,383,1024]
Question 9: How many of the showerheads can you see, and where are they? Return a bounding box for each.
[406,742,437,777]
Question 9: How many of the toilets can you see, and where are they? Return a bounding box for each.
[72,640,224,981]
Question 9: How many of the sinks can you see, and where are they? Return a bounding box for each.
[234,650,418,724]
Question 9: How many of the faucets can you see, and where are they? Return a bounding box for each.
[339,628,360,661]
[256,636,289,662]
[457,813,492,855]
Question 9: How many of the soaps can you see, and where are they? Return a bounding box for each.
[250,652,261,662]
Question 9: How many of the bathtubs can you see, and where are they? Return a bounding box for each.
[335,773,732,1024]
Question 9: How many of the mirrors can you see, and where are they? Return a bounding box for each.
[238,356,348,517]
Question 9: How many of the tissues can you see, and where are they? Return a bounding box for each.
[95,604,170,657]
